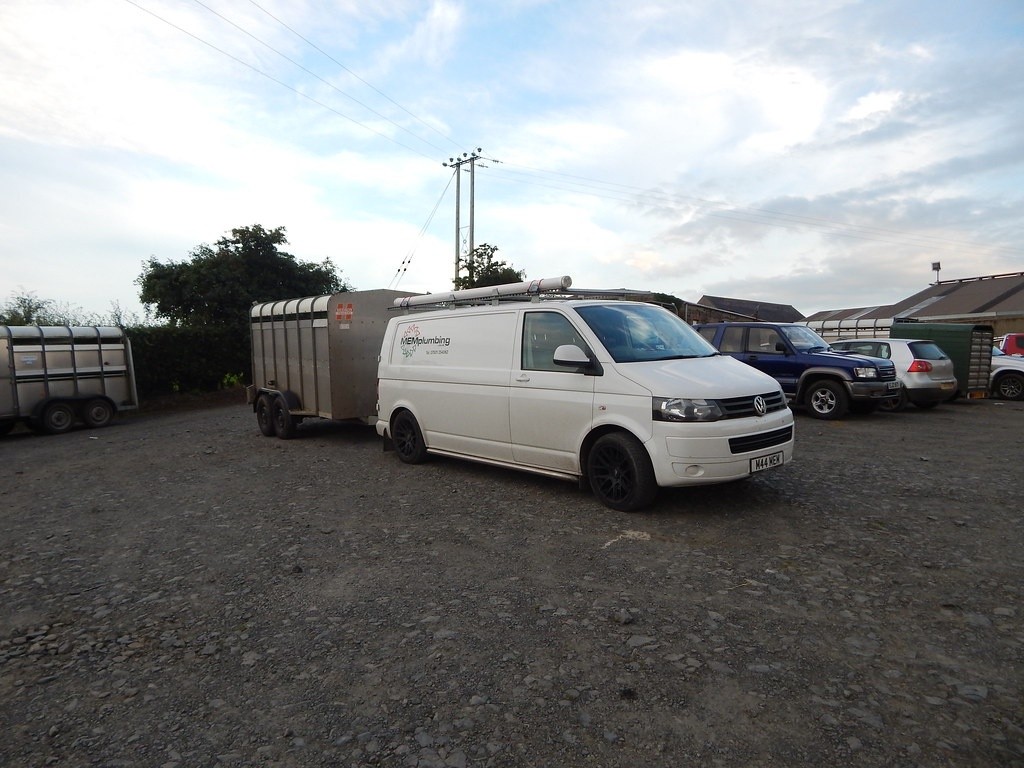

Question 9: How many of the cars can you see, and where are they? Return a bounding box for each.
[828,339,958,411]
[990,344,1024,402]
[993,333,1024,358]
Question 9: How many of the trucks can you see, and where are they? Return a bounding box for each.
[246,286,797,511]
[793,316,994,407]
[0,324,140,432]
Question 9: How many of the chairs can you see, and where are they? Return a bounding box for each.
[526,319,581,373]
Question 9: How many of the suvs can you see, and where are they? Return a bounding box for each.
[689,322,901,417]
